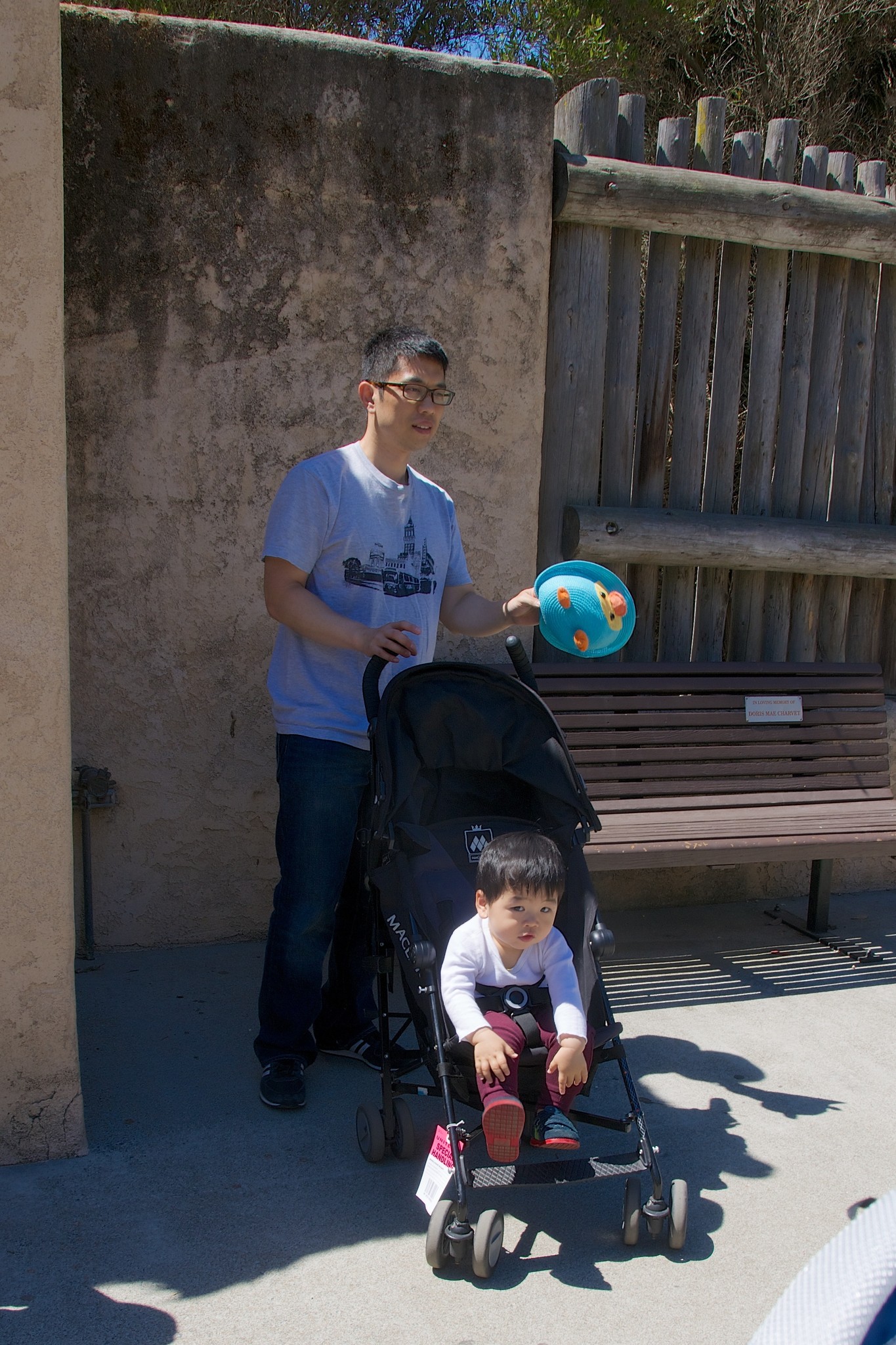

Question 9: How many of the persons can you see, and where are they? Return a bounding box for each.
[440,831,595,1163]
[251,320,540,1109]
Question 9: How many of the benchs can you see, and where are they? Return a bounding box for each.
[500,660,896,963]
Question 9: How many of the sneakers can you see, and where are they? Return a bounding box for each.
[529,1105,581,1150]
[480,1097,526,1162]
[259,1056,307,1110]
[319,1023,417,1071]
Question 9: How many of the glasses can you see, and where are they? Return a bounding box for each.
[368,383,456,406]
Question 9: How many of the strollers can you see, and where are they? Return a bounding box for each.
[363,630,688,1279]
